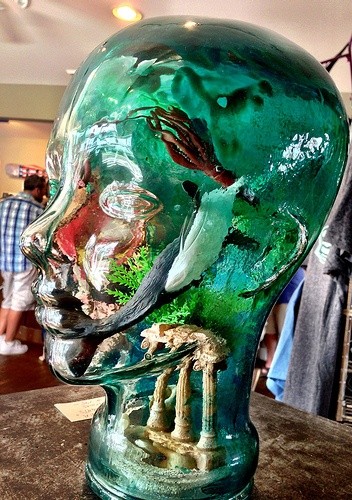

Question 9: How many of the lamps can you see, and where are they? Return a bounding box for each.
[112,4,143,24]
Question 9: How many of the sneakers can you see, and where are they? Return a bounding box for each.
[1,334,29,356]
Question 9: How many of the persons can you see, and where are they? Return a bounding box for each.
[20,14,349,499]
[0,174,48,356]
[263,265,308,371]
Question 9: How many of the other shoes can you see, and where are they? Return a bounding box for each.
[258,364,278,378]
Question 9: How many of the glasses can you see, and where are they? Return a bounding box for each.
[35,175,46,191]
